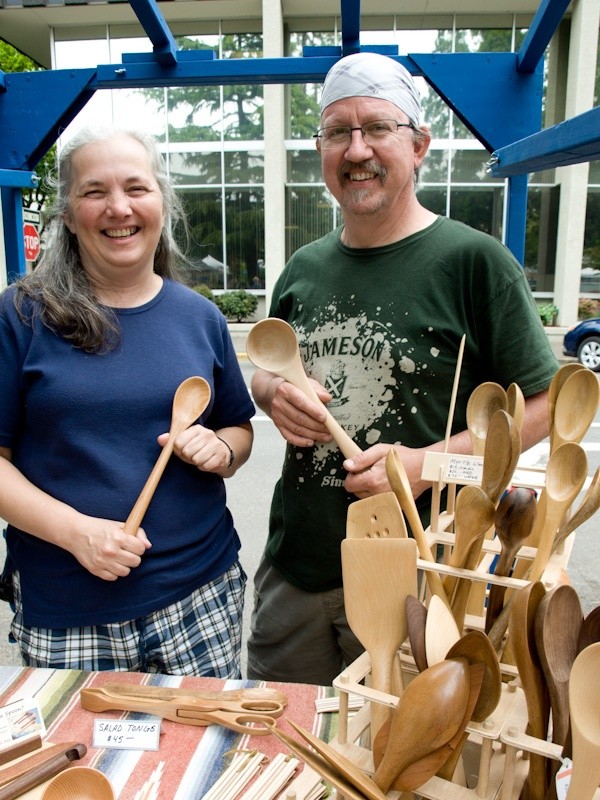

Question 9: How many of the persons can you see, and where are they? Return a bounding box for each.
[246,52,564,689]
[0,124,254,683]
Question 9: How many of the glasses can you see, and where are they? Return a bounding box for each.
[313,118,414,152]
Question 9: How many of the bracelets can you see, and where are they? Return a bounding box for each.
[217,437,235,468]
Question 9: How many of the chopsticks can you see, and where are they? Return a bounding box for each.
[200,750,328,800]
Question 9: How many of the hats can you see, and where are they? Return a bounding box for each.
[320,52,423,126]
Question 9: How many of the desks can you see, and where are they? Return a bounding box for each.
[0,665,599,800]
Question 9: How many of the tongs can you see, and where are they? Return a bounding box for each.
[81,686,283,737]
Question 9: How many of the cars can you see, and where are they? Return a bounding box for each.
[562,317,600,372]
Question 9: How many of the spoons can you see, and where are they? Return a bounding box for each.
[123,376,211,535]
[372,362,600,800]
[101,680,291,711]
